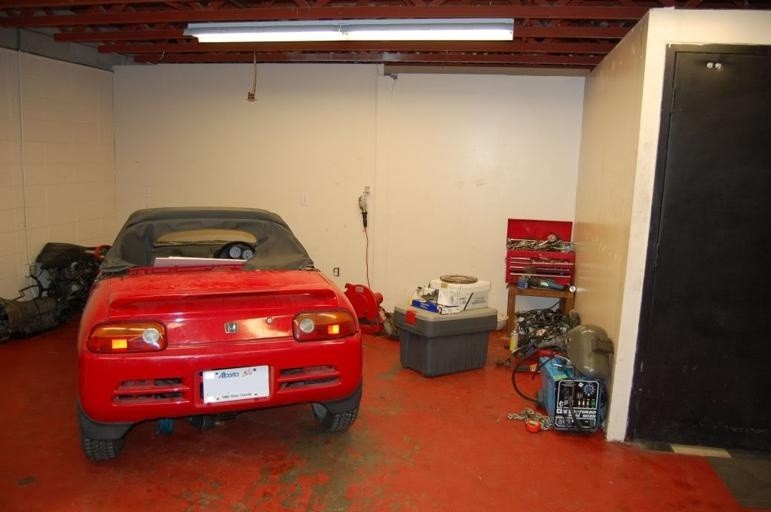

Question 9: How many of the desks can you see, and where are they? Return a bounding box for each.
[505,286,575,348]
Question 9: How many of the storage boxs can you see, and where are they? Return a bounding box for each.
[394,306,497,378]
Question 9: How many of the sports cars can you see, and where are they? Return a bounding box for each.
[75,206,364,464]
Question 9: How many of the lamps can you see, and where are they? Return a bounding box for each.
[182,19,514,43]
